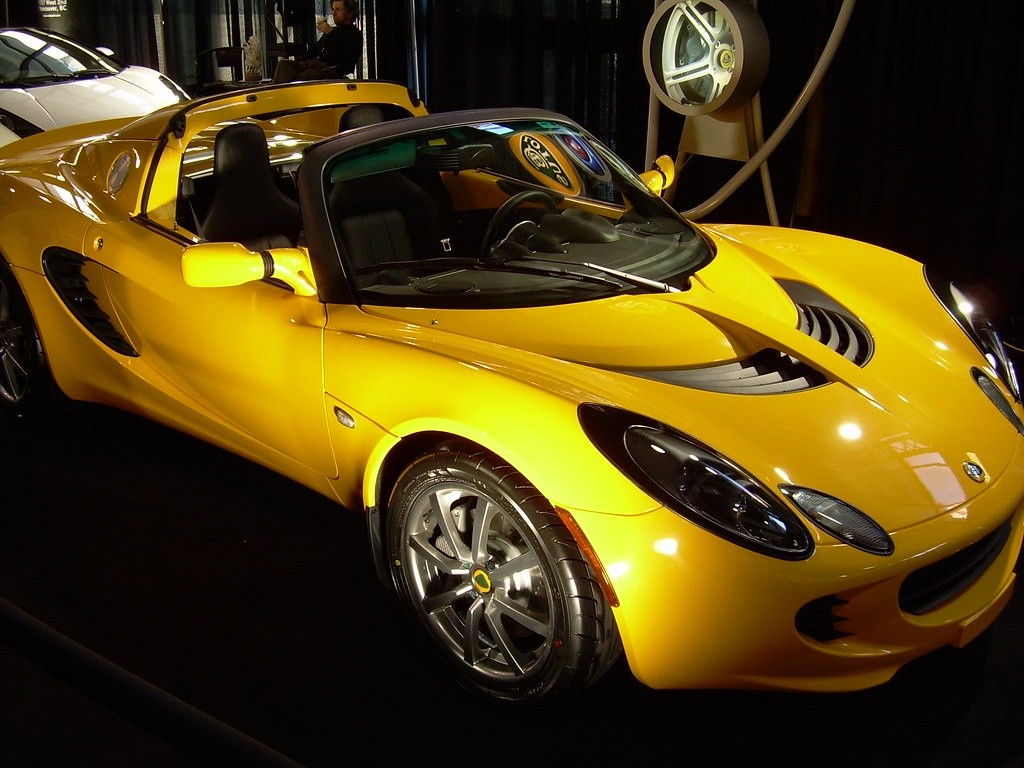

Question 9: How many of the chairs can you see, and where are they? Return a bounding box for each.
[327,99,437,256]
[189,120,302,250]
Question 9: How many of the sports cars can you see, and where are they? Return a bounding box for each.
[0,26,191,146]
[0,78,1023,707]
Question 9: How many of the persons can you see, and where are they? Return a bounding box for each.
[274,0,363,84]
